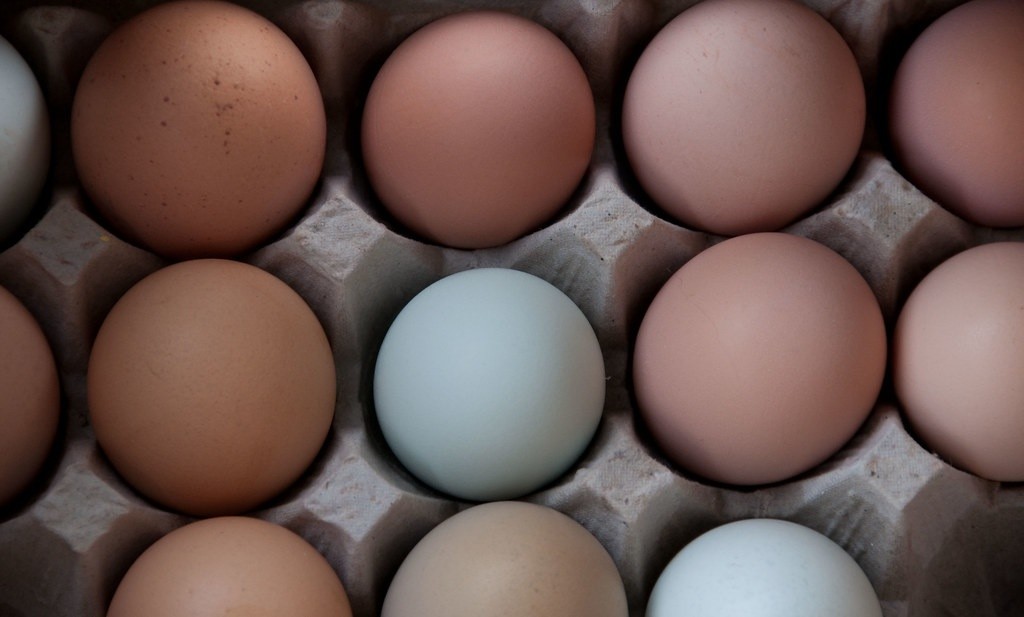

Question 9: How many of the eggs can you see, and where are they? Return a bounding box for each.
[0,0,1024,617]
